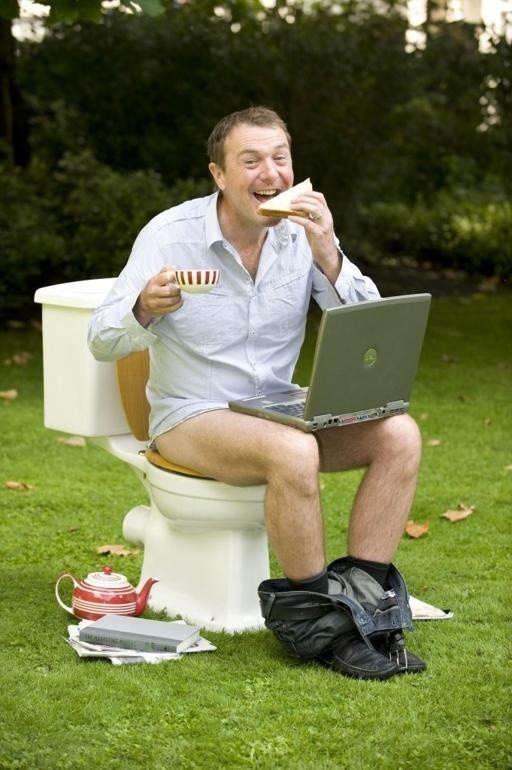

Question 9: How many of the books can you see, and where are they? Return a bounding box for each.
[79,614,200,653]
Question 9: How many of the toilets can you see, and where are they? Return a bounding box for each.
[31,277,274,634]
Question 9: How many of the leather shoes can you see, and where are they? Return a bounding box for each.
[310,628,427,681]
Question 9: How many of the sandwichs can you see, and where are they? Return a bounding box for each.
[257,176,313,218]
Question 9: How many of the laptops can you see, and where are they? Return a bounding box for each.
[228,292,432,433]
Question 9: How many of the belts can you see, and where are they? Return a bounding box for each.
[266,604,335,621]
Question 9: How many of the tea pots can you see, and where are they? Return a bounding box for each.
[56,566,159,623]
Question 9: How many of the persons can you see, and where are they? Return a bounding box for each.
[87,106,423,680]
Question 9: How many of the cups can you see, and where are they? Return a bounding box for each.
[168,270,219,293]
